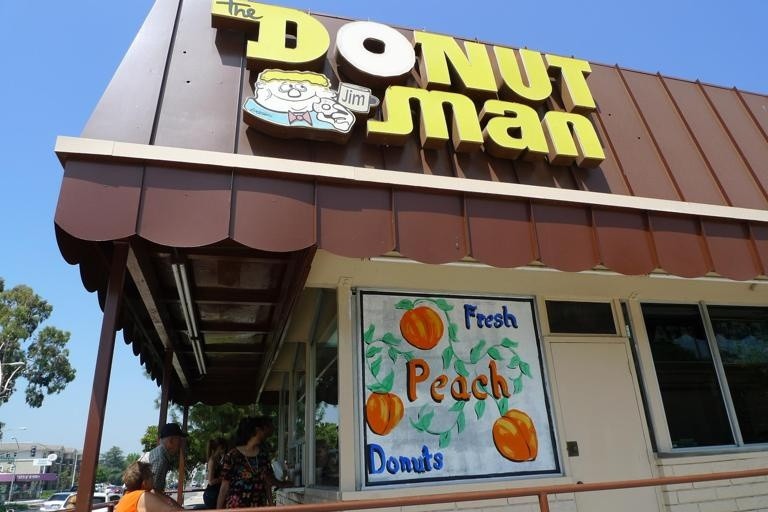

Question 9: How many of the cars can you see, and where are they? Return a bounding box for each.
[167,480,178,490]
[39,482,123,512]
[192,481,200,487]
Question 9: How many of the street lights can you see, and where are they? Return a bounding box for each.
[8,438,19,501]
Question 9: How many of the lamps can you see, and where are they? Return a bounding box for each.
[172,258,207,375]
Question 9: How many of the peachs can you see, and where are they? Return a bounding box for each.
[492,409,538,461]
[367,393,404,436]
[400,307,444,350]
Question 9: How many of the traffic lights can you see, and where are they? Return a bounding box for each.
[31,447,36,456]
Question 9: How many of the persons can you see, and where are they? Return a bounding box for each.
[273,477,290,488]
[317,439,335,467]
[123,423,189,493]
[216,414,275,509]
[113,461,179,512]
[203,438,227,506]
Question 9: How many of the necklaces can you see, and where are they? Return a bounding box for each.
[242,445,259,474]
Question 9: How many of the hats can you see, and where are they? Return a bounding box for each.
[159,423,184,439]
